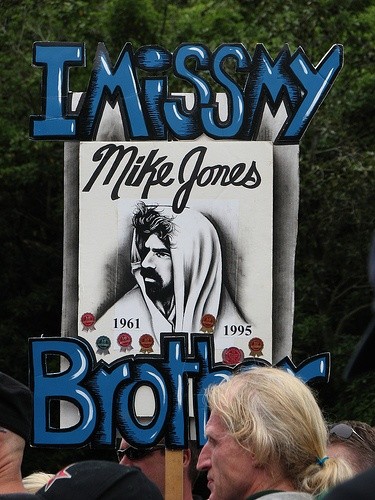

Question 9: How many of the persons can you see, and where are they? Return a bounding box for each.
[195,367,352,500]
[327,421,375,483]
[0,371,195,500]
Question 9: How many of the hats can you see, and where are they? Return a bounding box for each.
[0,461,164,500]
[0,373,33,443]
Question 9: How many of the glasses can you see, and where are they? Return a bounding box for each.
[329,424,366,442]
[117,444,165,461]
